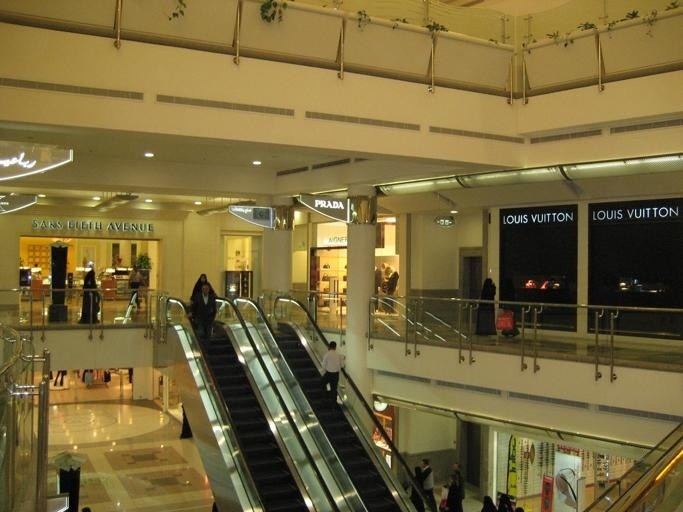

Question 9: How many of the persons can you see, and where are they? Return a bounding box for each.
[54,370,66,386]
[189,274,214,310]
[376,263,399,312]
[409,459,524,512]
[319,341,345,409]
[128,264,147,309]
[194,283,217,340]
